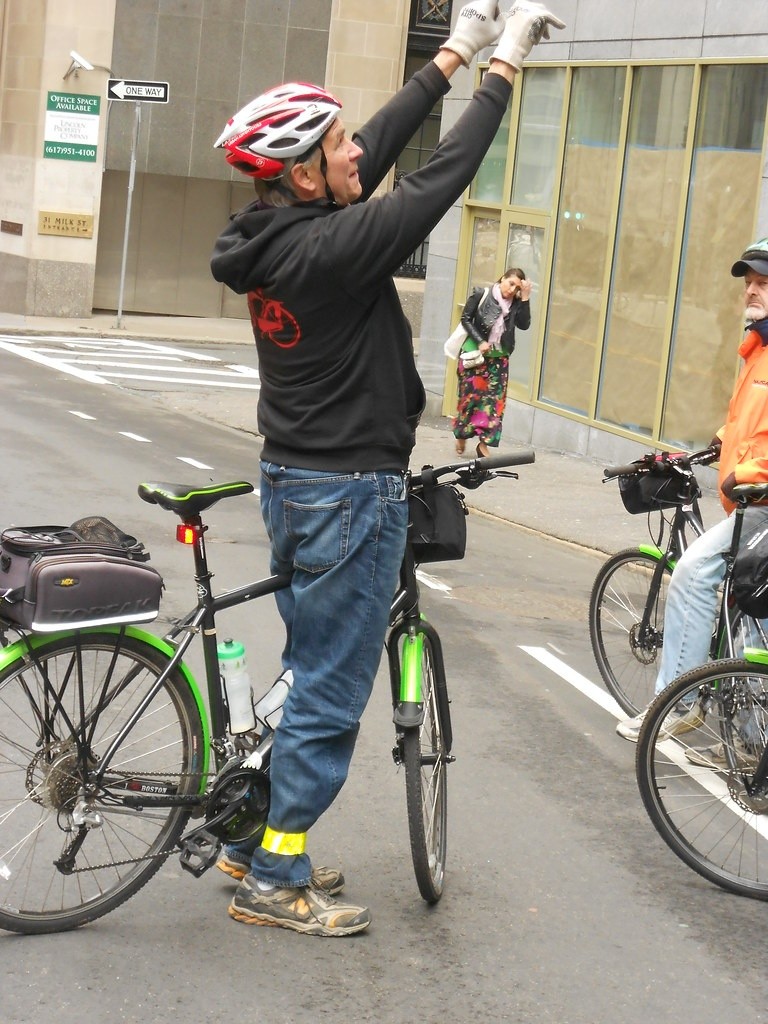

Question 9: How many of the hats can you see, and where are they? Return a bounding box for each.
[731,258,768,277]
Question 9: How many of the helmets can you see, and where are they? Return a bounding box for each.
[213,81,343,186]
[741,236,768,260]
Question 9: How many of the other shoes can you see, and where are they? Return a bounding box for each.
[476,443,485,458]
[456,443,465,453]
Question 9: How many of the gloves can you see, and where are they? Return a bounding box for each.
[720,471,739,502]
[489,1,566,73]
[702,435,722,465]
[438,0,506,73]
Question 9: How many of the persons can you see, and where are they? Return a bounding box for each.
[219,2,568,940]
[614,238,768,772]
[455,268,533,458]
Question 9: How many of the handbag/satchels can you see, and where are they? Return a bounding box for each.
[0,513,167,640]
[443,287,489,361]
[459,349,485,369]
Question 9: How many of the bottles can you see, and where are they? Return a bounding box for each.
[217,638,256,735]
[253,670,296,726]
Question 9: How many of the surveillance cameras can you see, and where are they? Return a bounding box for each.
[71,51,95,71]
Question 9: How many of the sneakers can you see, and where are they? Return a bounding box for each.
[683,737,759,776]
[615,700,705,745]
[226,873,373,936]
[215,853,346,896]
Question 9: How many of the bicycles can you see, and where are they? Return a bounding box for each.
[635,645,768,901]
[0,451,535,935]
[589,444,768,780]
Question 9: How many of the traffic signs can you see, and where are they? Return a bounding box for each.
[106,79,170,105]
